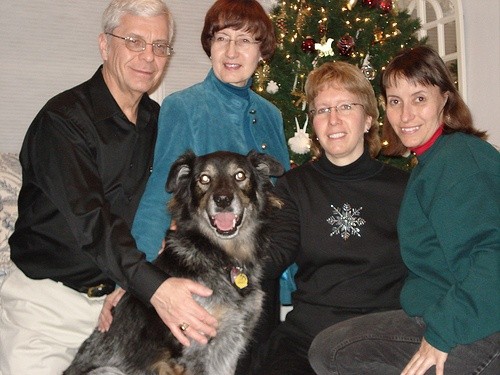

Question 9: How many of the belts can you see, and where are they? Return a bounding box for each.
[50,279,115,297]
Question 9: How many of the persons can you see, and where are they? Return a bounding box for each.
[98,0,298,335]
[1,0,219,375]
[308,43,500,375]
[255,61,412,374]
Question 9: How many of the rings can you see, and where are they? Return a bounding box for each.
[180,323,190,331]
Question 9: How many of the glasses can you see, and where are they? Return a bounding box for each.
[105,33,173,57]
[311,102,364,119]
[211,33,261,47]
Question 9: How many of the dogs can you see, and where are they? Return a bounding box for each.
[60,147,285,374]
